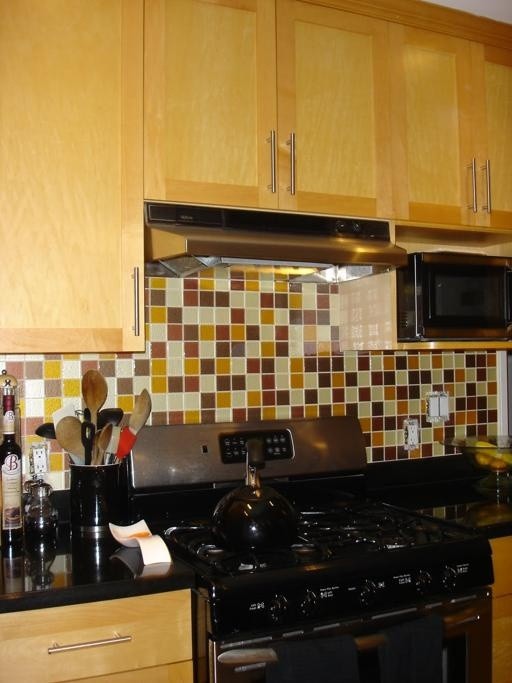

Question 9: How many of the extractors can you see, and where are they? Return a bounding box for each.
[143,203,408,282]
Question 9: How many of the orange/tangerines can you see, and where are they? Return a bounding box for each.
[475,453,492,465]
[490,461,507,469]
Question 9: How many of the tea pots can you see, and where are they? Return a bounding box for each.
[209,436,305,551]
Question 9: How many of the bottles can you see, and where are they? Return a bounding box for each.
[0,367,24,542]
[2,542,25,599]
[24,476,61,533]
[25,536,60,587]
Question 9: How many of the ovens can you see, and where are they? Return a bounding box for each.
[213,588,491,683]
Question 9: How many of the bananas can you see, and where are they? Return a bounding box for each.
[476,441,512,464]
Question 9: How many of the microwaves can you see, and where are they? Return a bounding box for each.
[393,249,510,344]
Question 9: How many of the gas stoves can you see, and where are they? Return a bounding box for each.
[151,500,495,630]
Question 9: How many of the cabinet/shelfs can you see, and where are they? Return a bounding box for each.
[0,583,195,680]
[481,535,512,682]
[338,1,512,353]
[0,0,151,354]
[142,1,394,220]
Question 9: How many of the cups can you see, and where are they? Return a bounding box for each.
[70,527,124,583]
[68,461,125,530]
